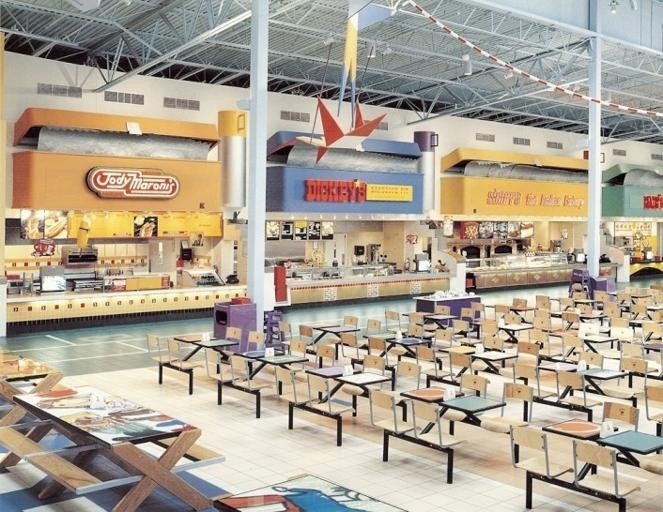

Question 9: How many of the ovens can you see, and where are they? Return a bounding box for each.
[62,247,98,266]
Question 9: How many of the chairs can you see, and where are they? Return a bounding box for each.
[146,284,663,512]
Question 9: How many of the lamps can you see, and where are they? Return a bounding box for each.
[503,71,514,80]
[463,60,472,76]
[628,0,638,11]
[322,36,335,46]
[366,43,376,59]
[607,0,620,14]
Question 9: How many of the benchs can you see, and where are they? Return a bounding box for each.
[0,348,225,512]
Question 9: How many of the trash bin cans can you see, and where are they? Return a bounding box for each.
[213,302,256,352]
